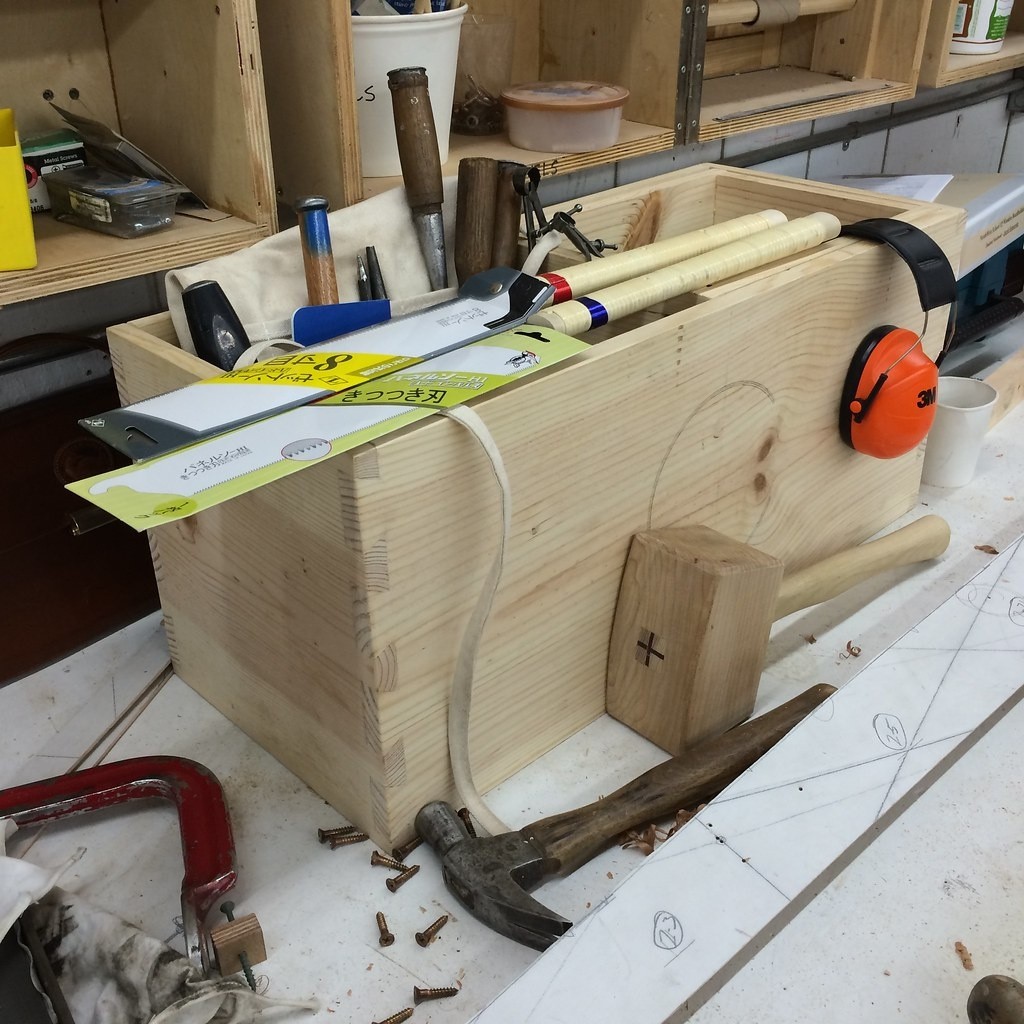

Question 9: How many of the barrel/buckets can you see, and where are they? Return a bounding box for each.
[948,0,1015,55]
[352,1,469,178]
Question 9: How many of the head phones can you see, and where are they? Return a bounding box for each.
[838,217,958,458]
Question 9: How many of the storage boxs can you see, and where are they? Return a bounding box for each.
[20,126,89,213]
[1,109,39,270]
[43,170,186,241]
[105,161,964,859]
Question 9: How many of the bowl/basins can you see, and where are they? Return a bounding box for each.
[499,79,630,154]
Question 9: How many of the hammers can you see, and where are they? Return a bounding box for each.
[413,684,842,956]
[597,517,951,756]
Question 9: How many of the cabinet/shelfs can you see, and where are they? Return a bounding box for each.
[255,1,932,230]
[918,0,1024,89]
[0,0,281,306]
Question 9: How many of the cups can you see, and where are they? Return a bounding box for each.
[920,376,1002,491]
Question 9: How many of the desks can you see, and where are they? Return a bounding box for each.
[0,290,1024,1024]
